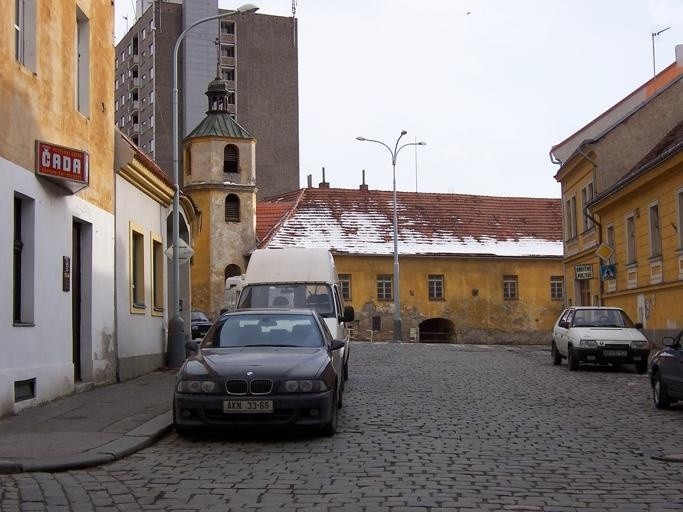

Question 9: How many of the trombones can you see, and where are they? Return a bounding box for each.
[600,265,615,281]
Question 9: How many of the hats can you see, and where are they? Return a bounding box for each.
[172,308,344,436]
[649,330,682,409]
[189,308,214,340]
[552,305,650,375]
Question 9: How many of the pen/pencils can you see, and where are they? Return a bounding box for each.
[237,247,350,380]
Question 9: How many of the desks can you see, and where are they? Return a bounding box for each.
[354,131,426,341]
[167,3,259,366]
